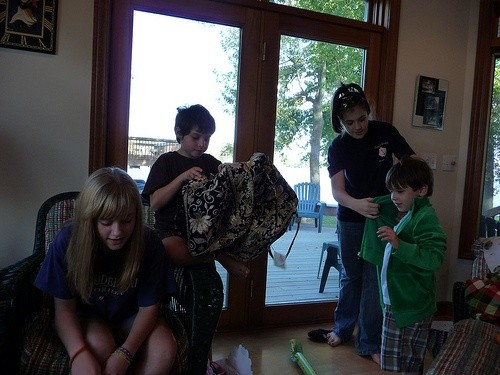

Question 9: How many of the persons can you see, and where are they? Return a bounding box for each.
[358,158,448,375]
[324,84,421,366]
[34,168,178,375]
[141,104,250,375]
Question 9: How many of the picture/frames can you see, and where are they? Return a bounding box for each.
[0,0,59,55]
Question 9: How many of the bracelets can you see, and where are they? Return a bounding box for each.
[69,347,88,370]
[111,347,132,365]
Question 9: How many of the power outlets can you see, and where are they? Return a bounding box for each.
[421,153,436,170]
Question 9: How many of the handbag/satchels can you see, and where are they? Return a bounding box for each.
[181,151,301,264]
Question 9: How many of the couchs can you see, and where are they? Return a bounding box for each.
[424,237,500,375]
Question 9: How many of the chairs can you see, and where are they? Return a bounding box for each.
[317,220,343,295]
[0,191,223,375]
[289,182,326,233]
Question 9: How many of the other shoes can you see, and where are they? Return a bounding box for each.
[308,329,333,343]
[427,320,454,358]
